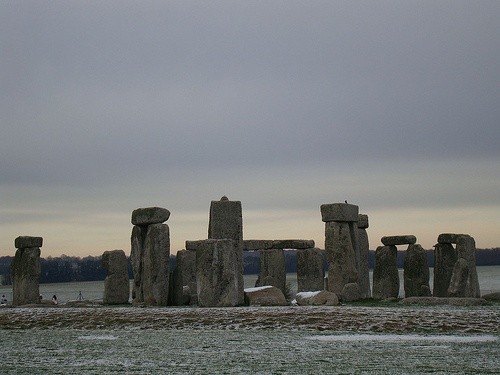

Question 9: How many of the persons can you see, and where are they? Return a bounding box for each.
[52,295,58,305]
[1,295,6,304]
